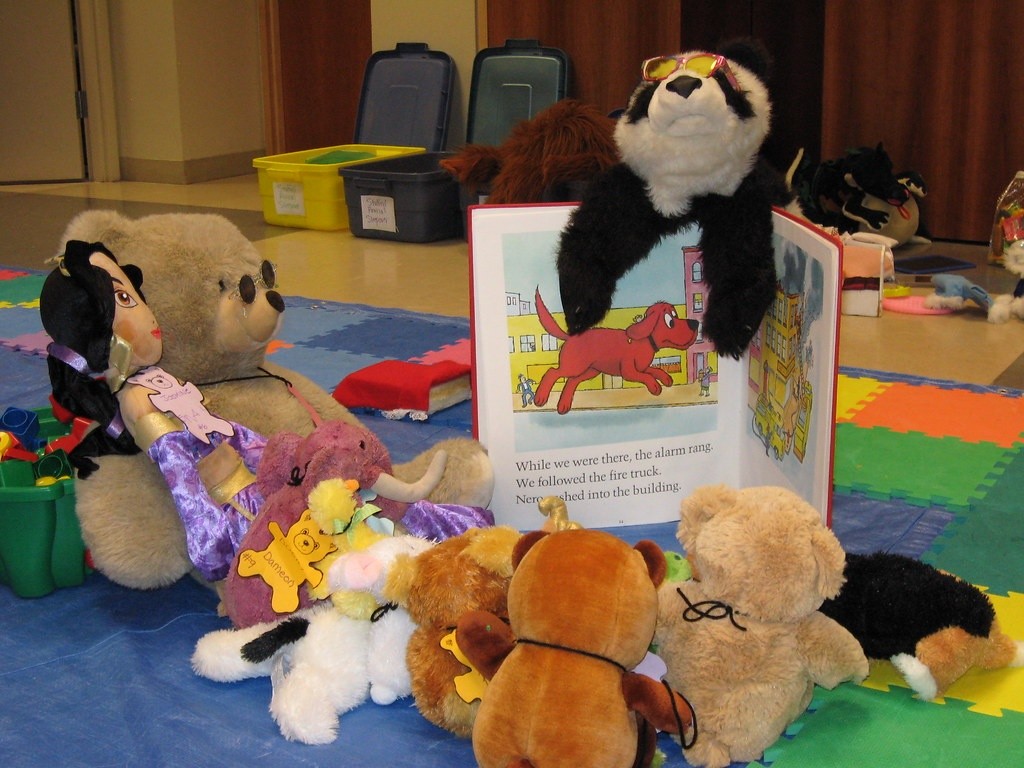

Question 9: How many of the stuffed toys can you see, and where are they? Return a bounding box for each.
[35,211,1019,768]
[441,49,1024,362]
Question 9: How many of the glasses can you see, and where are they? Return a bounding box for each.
[641,56,741,95]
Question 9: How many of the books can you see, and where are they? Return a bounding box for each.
[466,200,844,537]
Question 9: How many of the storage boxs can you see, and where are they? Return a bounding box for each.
[253,144,478,242]
[0,405,87,597]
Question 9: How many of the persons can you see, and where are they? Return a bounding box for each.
[40,240,495,583]
